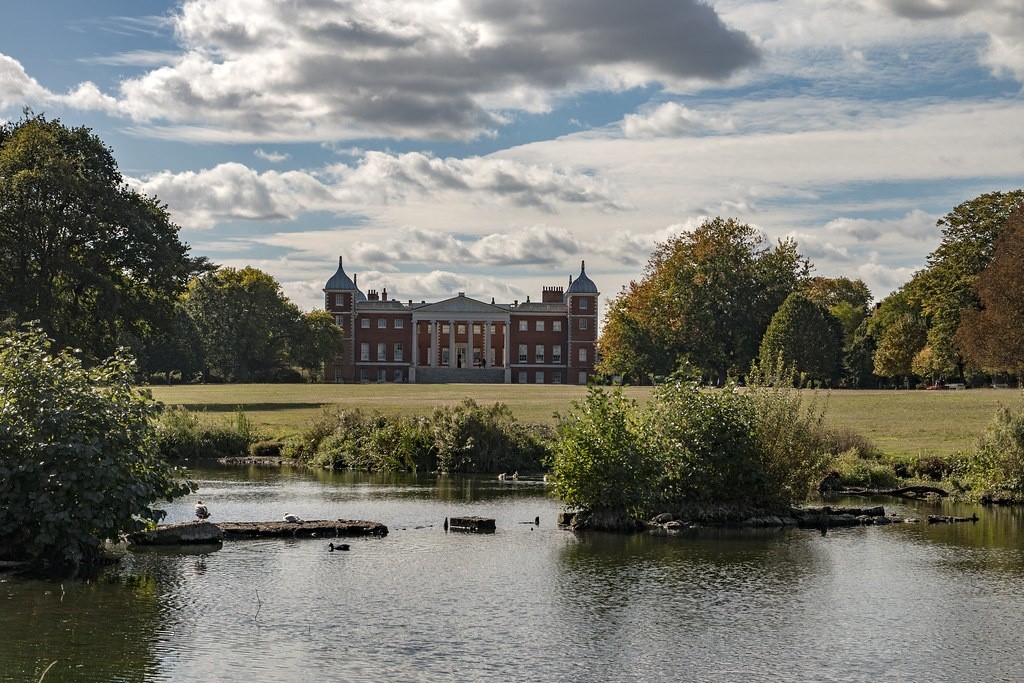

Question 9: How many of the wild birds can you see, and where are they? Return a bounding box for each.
[328,543,353,552]
[197,501,211,518]
[283,512,305,524]
